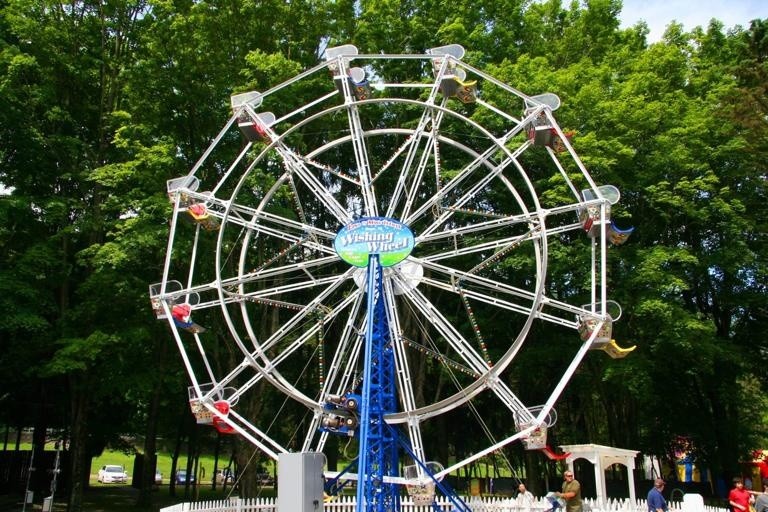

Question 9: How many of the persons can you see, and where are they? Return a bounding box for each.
[728,477,756,512]
[647,478,670,512]
[755,480,768,512]
[550,469,583,512]
[216,470,224,485]
[516,483,534,502]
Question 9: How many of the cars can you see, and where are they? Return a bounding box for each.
[172,468,195,486]
[255,465,274,486]
[96,465,127,484]
[213,469,234,485]
[154,469,164,485]
